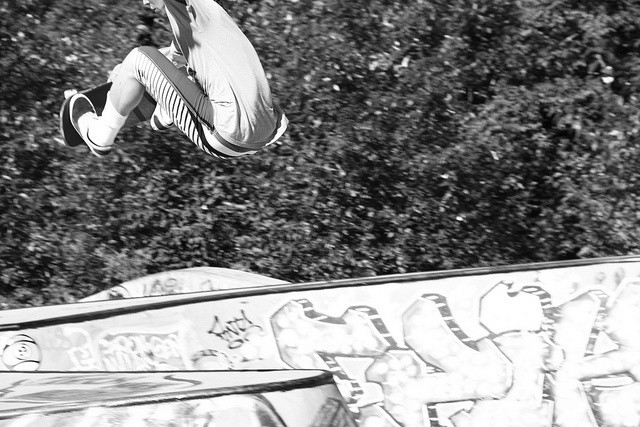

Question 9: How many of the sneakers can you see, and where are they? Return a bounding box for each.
[69,93,115,158]
[150,102,174,131]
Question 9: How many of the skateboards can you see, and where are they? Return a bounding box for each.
[59,82,157,151]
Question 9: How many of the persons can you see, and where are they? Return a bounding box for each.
[68,0,289,161]
